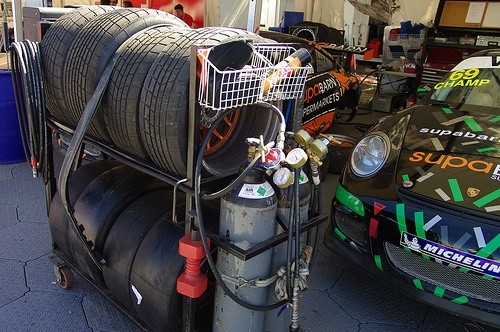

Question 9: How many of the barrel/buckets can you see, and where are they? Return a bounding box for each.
[0,69,28,165]
[319,132,359,176]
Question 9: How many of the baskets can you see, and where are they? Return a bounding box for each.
[197,42,271,111]
[251,42,312,101]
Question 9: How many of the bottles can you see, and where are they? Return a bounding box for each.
[259,47,312,93]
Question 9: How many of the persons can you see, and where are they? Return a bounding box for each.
[174,4,195,29]
[100,0,133,7]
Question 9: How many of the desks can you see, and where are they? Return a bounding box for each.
[373,70,416,113]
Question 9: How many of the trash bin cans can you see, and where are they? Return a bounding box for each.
[0,68,30,164]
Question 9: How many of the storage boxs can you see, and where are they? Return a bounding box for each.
[480,1,500,29]
[282,11,305,28]
[438,0,488,28]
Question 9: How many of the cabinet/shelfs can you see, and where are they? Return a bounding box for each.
[415,0,500,94]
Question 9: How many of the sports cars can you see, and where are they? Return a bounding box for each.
[322,46,500,330]
